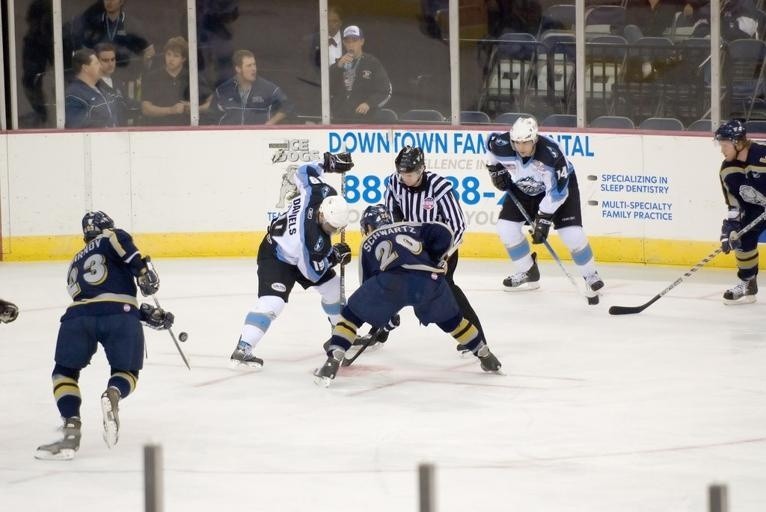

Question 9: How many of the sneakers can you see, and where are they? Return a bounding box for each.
[231,333,263,367]
[36,416,80,454]
[503,253,539,287]
[724,278,758,299]
[477,346,501,371]
[315,349,345,380]
[584,266,604,291]
[102,387,120,446]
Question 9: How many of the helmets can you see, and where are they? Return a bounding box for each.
[713,121,746,145]
[360,203,392,232]
[319,195,350,230]
[395,147,425,186]
[83,211,115,239]
[507,118,539,153]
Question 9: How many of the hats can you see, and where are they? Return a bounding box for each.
[343,26,361,43]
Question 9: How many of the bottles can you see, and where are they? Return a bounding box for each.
[343,49,354,71]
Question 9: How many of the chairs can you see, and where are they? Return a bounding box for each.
[476,1,766,130]
[402,109,443,123]
[446,109,490,124]
[378,107,399,123]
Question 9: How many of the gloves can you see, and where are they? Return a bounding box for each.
[529,214,552,244]
[332,243,353,265]
[323,152,353,174]
[487,163,511,191]
[138,257,160,296]
[721,219,742,254]
[140,304,175,329]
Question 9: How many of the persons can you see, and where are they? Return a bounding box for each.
[0,297,21,328]
[63,1,394,128]
[371,145,486,351]
[609,0,765,85]
[311,201,501,380]
[229,150,355,364]
[32,208,172,455]
[715,108,763,301]
[482,111,606,293]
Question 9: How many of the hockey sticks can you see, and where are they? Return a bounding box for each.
[504,188,600,305]
[340,171,376,346]
[609,213,766,316]
[341,327,384,368]
